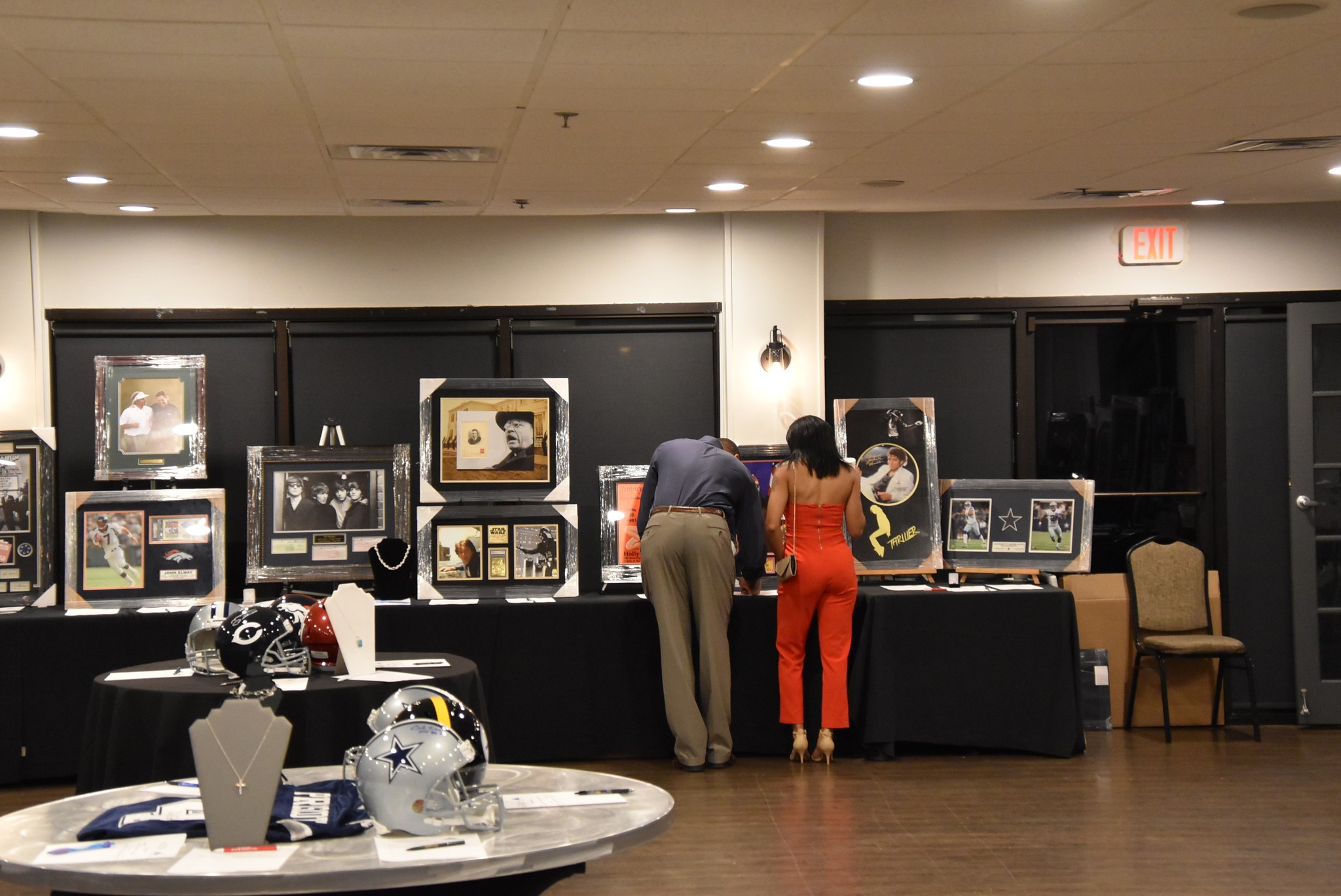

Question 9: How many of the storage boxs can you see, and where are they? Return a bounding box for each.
[1063,570,1225,727]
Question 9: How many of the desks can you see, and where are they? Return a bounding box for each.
[1,577,864,787]
[1,765,675,894]
[77,653,494,795]
[856,582,1087,757]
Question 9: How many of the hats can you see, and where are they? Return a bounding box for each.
[496,412,534,431]
[891,410,901,419]
[132,392,149,404]
[541,526,552,538]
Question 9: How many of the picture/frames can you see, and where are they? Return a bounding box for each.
[245,443,410,584]
[0,427,55,606]
[834,397,943,570]
[94,354,207,480]
[939,478,1095,572]
[735,445,790,590]
[420,378,570,503]
[417,504,579,600]
[64,488,226,609]
[598,465,650,584]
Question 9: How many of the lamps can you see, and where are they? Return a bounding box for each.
[761,326,789,374]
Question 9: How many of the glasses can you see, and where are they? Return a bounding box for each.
[502,421,525,429]
[288,482,302,487]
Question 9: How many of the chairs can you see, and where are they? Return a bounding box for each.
[1126,534,1261,744]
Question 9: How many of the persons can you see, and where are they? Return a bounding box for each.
[1039,501,1063,550]
[766,416,866,765]
[282,475,371,531]
[1,488,28,531]
[89,516,140,586]
[486,411,535,472]
[860,447,914,503]
[885,410,923,440]
[119,392,182,454]
[636,433,766,772]
[468,429,481,445]
[516,526,558,577]
[955,501,986,547]
[439,538,480,578]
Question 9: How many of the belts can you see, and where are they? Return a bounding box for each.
[651,506,727,518]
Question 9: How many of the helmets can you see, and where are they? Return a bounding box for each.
[965,501,971,507]
[1050,502,1057,507]
[344,686,488,802]
[355,719,504,835]
[96,516,108,529]
[184,594,340,674]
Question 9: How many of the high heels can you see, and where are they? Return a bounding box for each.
[811,729,834,765]
[789,729,810,764]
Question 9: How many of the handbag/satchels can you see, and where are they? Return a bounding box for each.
[775,555,797,583]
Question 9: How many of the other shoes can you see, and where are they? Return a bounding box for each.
[981,542,986,547]
[706,754,736,769]
[130,580,135,586]
[1056,546,1061,550]
[963,545,967,547]
[133,569,139,579]
[678,761,705,772]
[1052,537,1055,542]
